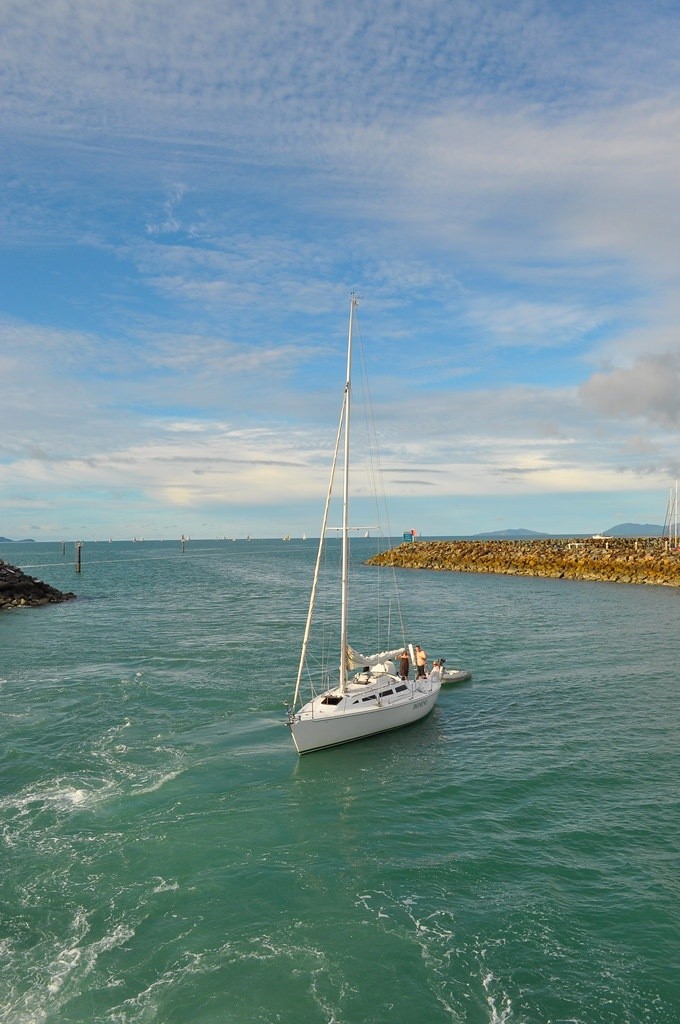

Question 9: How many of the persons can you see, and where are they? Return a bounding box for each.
[415,645,426,680]
[429,660,439,680]
[394,650,408,680]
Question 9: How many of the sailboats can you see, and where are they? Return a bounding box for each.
[289,292,443,756]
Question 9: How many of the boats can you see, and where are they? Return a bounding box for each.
[441,666,472,686]
[592,534,612,539]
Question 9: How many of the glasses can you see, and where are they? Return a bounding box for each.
[433,664,436,665]
